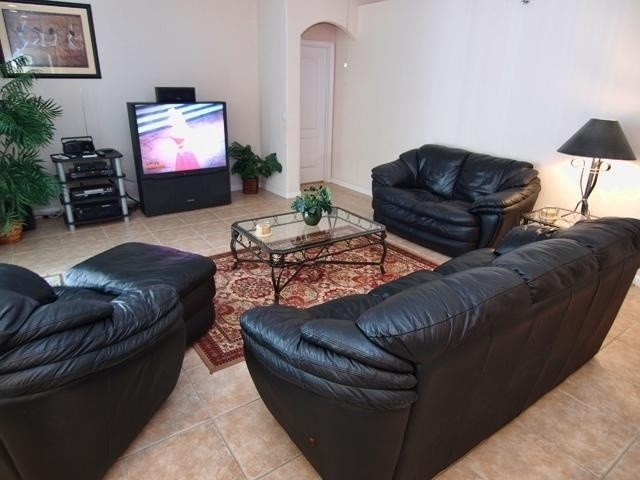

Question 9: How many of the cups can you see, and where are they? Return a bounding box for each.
[257,224,270,235]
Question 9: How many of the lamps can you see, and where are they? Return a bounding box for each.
[552,120,636,224]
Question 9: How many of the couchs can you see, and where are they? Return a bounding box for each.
[65,243,216,351]
[371,146,541,257]
[239,218,638,480]
[0,263,186,479]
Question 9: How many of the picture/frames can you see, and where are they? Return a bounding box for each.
[0,0,101,79]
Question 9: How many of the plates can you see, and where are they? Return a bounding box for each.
[255,233,272,237]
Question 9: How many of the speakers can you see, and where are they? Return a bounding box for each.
[155,86,196,102]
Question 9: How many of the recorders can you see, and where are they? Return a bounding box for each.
[61,136,95,154]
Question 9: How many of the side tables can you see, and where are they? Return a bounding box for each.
[522,206,598,227]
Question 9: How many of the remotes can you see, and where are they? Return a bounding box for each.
[96,150,105,156]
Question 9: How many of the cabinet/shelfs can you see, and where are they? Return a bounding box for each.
[137,170,232,217]
[50,149,131,232]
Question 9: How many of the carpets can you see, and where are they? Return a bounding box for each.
[193,225,440,373]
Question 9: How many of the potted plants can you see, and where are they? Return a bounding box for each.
[228,141,283,195]
[0,55,70,245]
[290,185,332,226]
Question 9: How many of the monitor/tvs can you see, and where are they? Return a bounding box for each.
[127,101,229,179]
